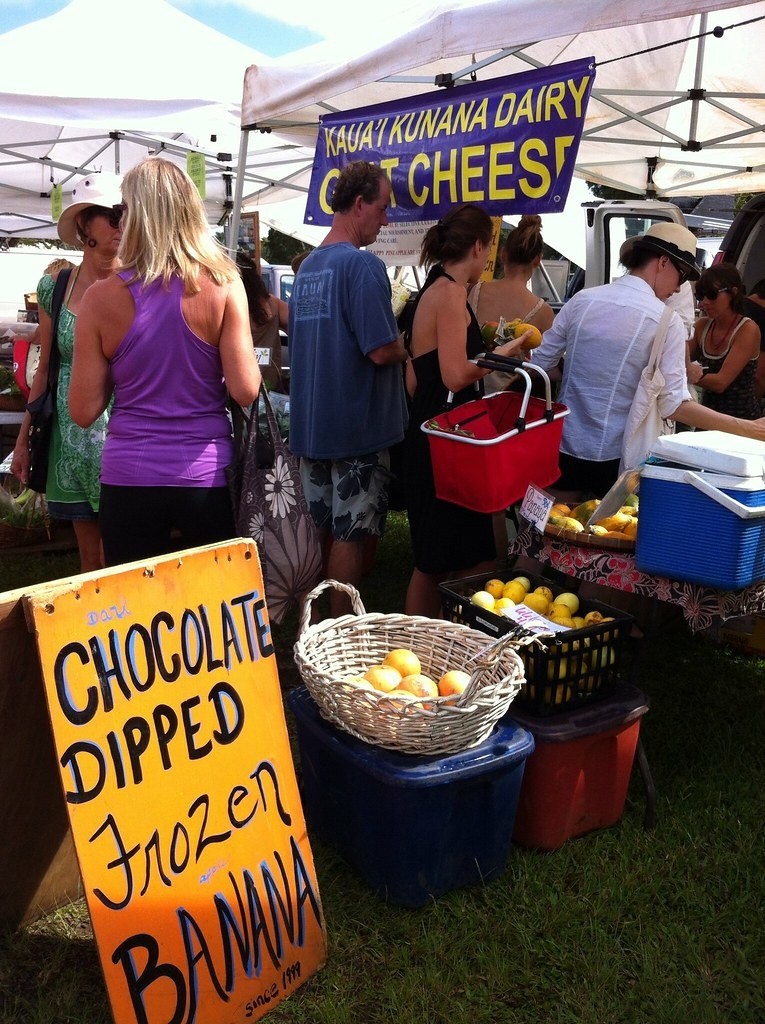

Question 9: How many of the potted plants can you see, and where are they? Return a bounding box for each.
[0,366,27,413]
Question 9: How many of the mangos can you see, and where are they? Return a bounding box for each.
[482,318,542,349]
[547,493,639,540]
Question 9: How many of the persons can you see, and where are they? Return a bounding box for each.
[397,203,533,619]
[67,157,262,568]
[465,215,554,396]
[10,172,128,573]
[287,160,408,619]
[528,222,765,492]
[685,263,761,432]
[226,252,289,393]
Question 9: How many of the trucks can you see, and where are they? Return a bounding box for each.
[0,247,295,366]
[385,195,765,318]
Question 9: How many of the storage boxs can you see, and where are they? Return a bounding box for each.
[436,566,636,720]
[0,322,40,357]
[286,686,536,911]
[419,390,572,514]
[633,429,765,591]
[24,292,38,310]
[507,678,652,853]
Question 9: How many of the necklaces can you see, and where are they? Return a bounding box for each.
[710,314,738,350]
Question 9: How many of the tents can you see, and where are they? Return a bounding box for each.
[0,0,315,325]
[228,0,765,263]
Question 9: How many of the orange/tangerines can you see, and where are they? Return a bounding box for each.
[340,649,472,718]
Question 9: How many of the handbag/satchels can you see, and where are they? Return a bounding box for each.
[228,380,325,626]
[23,268,71,494]
[618,305,677,475]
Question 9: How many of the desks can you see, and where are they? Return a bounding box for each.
[0,413,80,554]
[503,524,765,661]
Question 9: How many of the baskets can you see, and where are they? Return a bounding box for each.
[294,579,558,756]
[433,564,639,725]
[420,352,570,512]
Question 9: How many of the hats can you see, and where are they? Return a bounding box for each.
[620,222,701,281]
[57,173,126,248]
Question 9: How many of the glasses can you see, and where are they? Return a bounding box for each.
[689,285,734,302]
[82,208,122,229]
[658,251,690,286]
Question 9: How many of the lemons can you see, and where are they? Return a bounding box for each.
[453,576,618,704]
